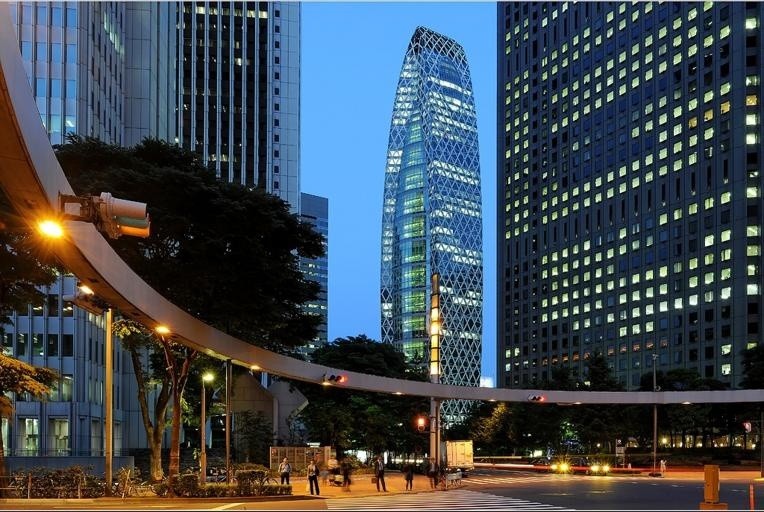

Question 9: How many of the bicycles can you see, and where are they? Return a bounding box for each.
[8,471,167,497]
[218,472,279,495]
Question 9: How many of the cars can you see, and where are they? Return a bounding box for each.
[529,458,563,473]
[566,457,607,475]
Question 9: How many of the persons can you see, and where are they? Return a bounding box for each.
[306,459,320,496]
[278,456,292,484]
[341,456,352,493]
[319,466,330,487]
[659,458,667,478]
[326,453,339,488]
[426,457,440,489]
[400,458,414,491]
[375,454,389,492]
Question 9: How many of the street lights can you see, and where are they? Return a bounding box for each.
[201,367,215,483]
[430,273,441,470]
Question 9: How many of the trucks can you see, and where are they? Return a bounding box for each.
[440,440,473,478]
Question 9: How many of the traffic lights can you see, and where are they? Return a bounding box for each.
[417,415,427,434]
[322,372,347,385]
[528,394,546,403]
[94,192,154,242]
